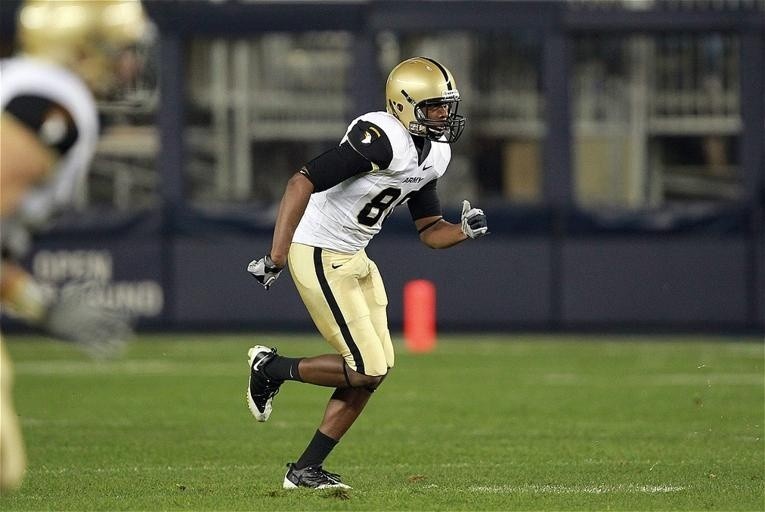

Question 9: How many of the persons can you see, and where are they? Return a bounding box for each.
[248,57,489,488]
[0,0,160,492]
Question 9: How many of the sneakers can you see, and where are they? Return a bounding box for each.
[281,462,355,493]
[246,344,284,423]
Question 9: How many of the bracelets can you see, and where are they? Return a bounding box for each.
[2,278,48,326]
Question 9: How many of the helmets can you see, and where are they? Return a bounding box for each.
[13,0,155,98]
[385,56,462,138]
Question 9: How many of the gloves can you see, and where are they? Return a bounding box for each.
[461,199,488,240]
[246,255,288,291]
